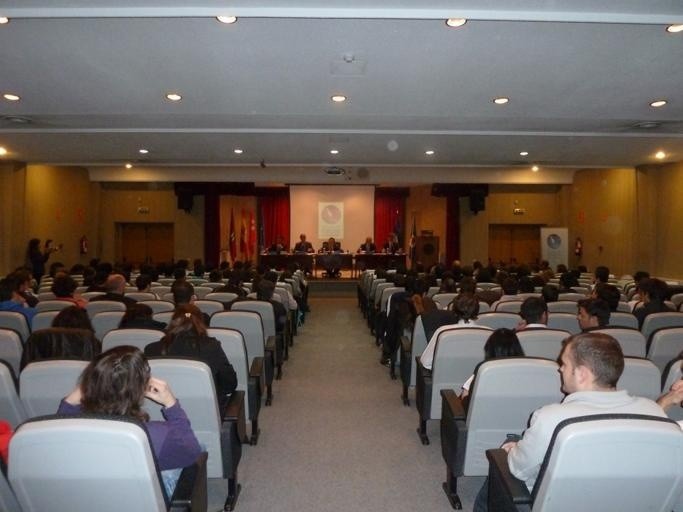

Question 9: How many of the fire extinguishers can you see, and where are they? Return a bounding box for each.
[80,234,88,254]
[574,237,582,254]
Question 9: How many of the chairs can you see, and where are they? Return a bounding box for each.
[353,264,682,512]
[0,247,307,511]
[321,242,342,278]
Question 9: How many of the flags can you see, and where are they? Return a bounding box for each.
[258,203,265,246]
[229,206,237,262]
[408,215,416,265]
[249,210,256,258]
[240,206,249,261]
[392,210,400,255]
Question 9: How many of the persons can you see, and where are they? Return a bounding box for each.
[356,237,683,511]
[318,238,344,278]
[0,233,313,471]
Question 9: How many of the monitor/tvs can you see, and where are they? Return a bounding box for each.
[421,230,432,236]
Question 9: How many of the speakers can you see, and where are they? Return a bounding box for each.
[469,192,486,211]
[177,194,192,210]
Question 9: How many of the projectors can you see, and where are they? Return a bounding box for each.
[327,168,341,175]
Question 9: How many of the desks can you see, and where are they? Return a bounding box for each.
[259,252,408,280]
